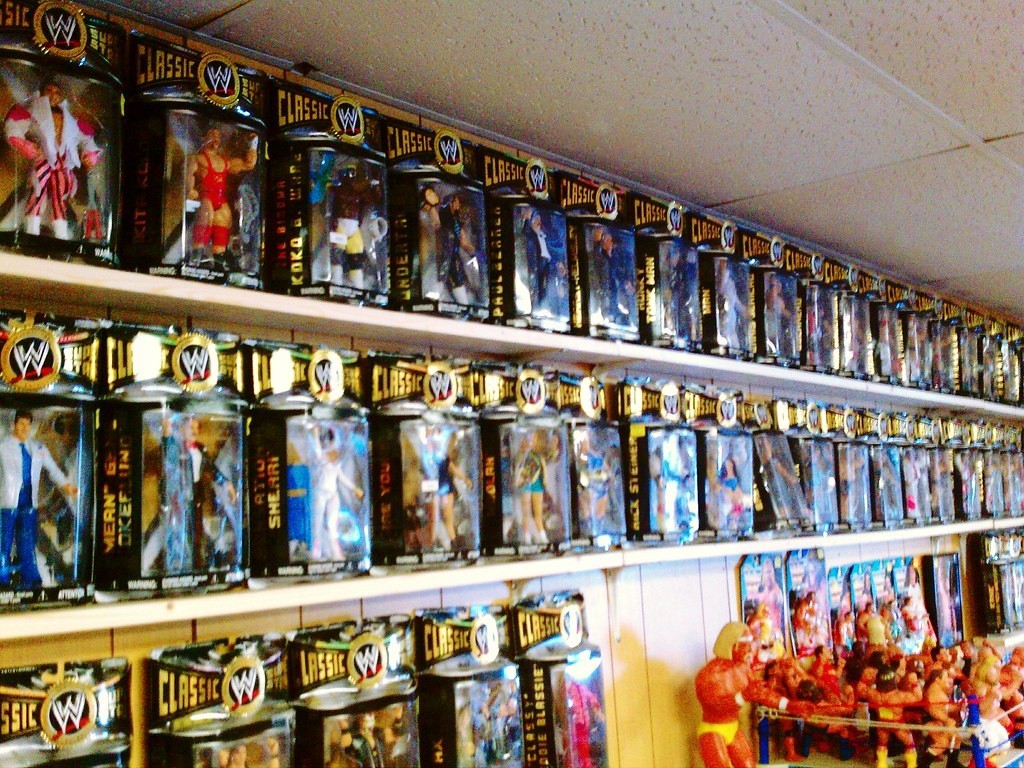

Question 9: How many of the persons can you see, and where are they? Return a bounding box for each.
[0,307,1024,593]
[695,623,816,768]
[0,61,1024,406]
[747,531,1024,768]
[218,673,608,768]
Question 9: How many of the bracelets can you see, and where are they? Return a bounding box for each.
[734,691,746,706]
[779,696,788,711]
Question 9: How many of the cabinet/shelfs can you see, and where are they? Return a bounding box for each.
[1,250,1022,648]
